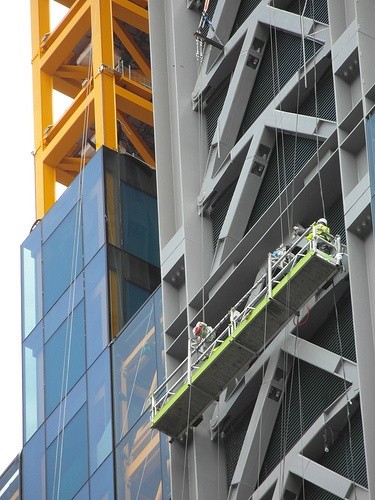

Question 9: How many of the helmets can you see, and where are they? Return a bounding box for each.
[317,218,327,226]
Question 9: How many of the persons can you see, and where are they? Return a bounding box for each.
[293,218,331,254]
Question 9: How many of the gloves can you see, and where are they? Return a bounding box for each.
[293,226,299,230]
